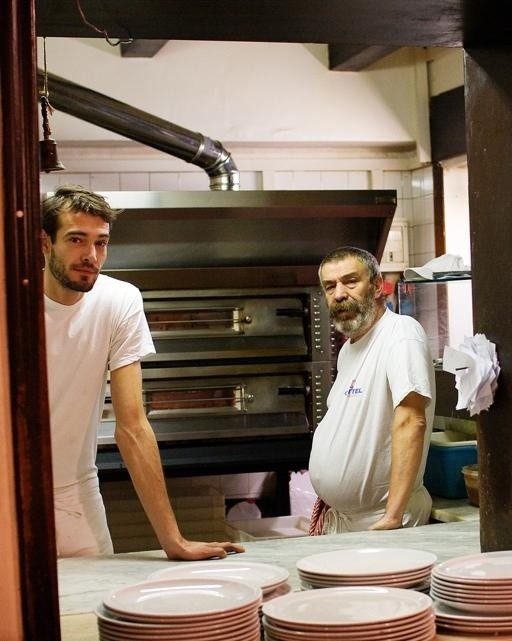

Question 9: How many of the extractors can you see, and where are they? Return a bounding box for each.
[36,189,398,286]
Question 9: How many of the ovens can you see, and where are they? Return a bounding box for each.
[93,291,338,474]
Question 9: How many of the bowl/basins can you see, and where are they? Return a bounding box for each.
[460,463,480,507]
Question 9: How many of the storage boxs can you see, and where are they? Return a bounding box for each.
[425,431,477,501]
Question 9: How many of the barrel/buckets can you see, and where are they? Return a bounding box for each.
[227,498,262,521]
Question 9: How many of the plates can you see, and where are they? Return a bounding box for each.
[296,547,439,594]
[146,559,292,603]
[430,550,512,636]
[259,586,437,641]
[94,576,263,641]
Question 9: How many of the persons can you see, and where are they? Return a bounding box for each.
[38,185,247,565]
[308,246,438,534]
